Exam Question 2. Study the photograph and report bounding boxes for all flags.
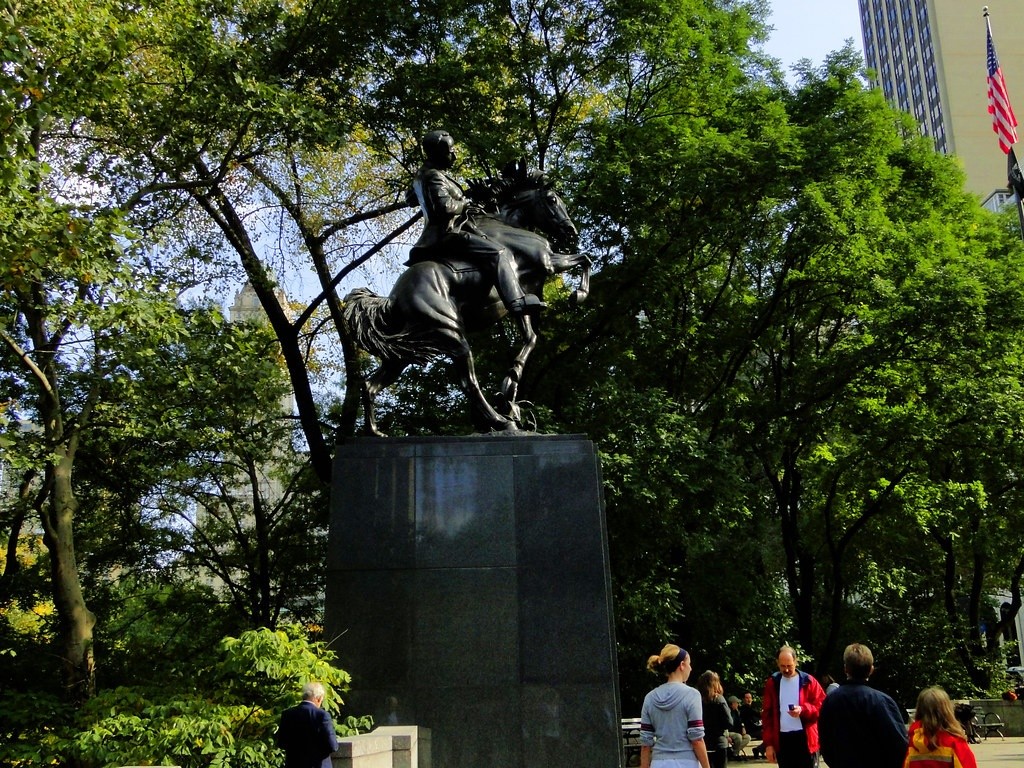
[987,26,1018,155]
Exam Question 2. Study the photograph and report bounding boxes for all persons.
[638,644,710,768]
[760,647,826,768]
[273,683,339,768]
[409,130,600,318]
[817,644,909,768]
[742,694,766,758]
[999,686,1024,701]
[697,670,733,768]
[954,700,981,744]
[903,688,977,768]
[822,674,839,695]
[728,696,751,756]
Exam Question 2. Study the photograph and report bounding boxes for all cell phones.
[789,705,794,710]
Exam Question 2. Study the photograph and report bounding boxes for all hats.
[728,695,742,703]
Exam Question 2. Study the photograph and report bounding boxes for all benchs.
[972,706,1005,744]
[621,717,641,768]
[906,708,916,722]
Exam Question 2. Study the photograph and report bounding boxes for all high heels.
[967,736,976,745]
[973,736,980,744]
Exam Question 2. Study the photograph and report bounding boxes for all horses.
[338,171,594,442]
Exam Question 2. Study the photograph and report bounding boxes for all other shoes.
[731,755,743,762]
[752,747,760,760]
[760,751,768,760]
